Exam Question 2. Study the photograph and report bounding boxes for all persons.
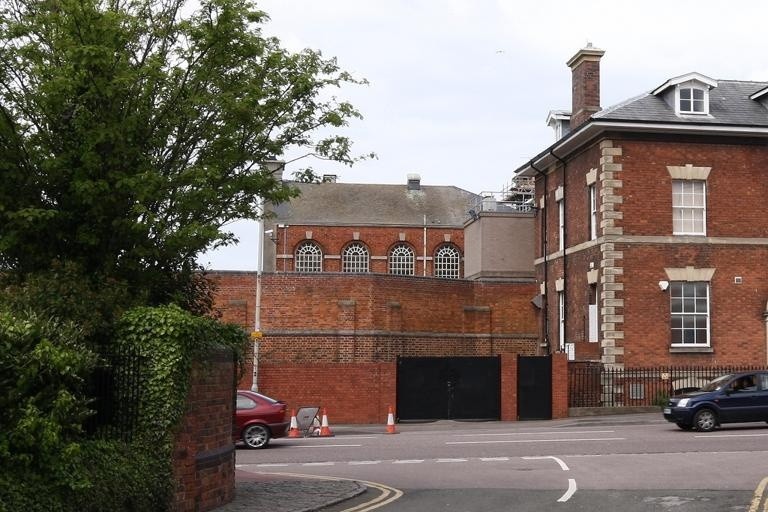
[740,376,752,388]
[731,380,739,389]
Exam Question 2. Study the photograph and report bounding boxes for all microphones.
[265,230,273,234]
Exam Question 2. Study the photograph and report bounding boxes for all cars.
[235,389,292,449]
[663,370,768,432]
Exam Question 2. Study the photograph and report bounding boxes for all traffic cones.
[313,411,320,432]
[385,406,400,434]
[288,408,300,437]
[318,407,334,437]
